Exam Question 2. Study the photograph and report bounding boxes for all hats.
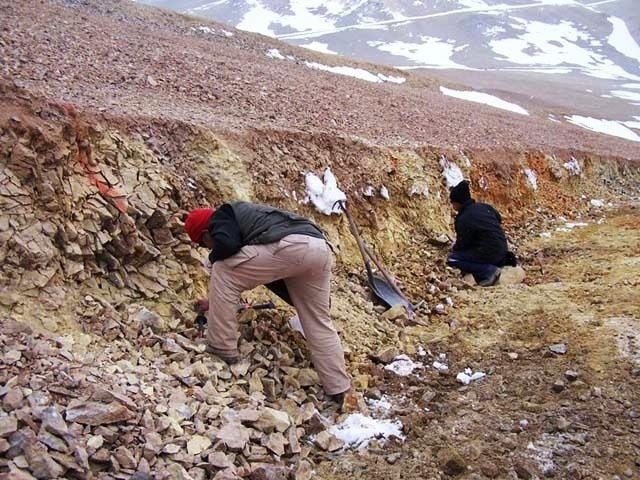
[450,181,473,204]
[185,208,214,243]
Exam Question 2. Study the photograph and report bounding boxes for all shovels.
[342,213,410,310]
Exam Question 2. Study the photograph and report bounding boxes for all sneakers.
[336,389,351,403]
[207,348,239,363]
[480,268,501,286]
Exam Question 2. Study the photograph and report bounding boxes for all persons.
[183,201,351,402]
[447,180,516,286]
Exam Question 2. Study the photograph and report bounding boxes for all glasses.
[198,235,208,248]
